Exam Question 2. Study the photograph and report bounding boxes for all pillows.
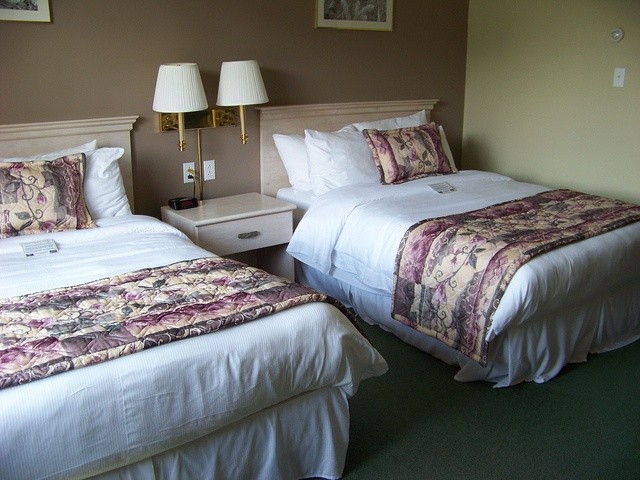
[270,125,370,189]
[3,138,100,162]
[82,146,134,227]
[0,153,97,239]
[437,126,458,174]
[361,123,463,182]
[353,108,427,134]
[303,128,385,191]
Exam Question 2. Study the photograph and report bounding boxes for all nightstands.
[160,191,296,286]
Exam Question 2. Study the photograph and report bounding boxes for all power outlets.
[182,162,196,185]
[202,159,216,181]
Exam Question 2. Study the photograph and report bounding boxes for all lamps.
[214,60,271,144]
[151,60,209,152]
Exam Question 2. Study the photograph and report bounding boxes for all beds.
[254,97,639,389]
[0,116,390,480]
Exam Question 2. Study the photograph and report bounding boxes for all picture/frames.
[0,0,52,25]
[311,0,395,32]
